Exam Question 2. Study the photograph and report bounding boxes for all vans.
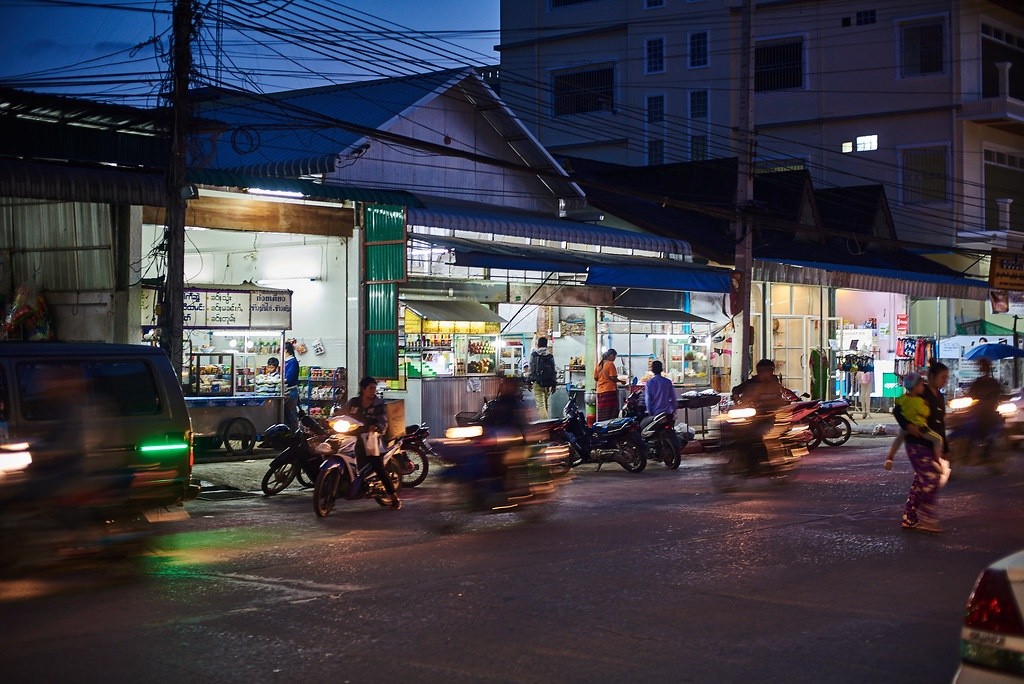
[0,338,193,520]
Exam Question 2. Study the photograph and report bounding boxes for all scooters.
[941,380,1009,460]
[725,398,827,470]
[454,393,581,478]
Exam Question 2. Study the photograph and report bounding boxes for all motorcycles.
[306,409,412,519]
[776,373,858,446]
[618,389,682,470]
[256,401,438,495]
[536,383,649,475]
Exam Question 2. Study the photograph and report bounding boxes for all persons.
[855,343,872,419]
[334,378,402,510]
[731,358,781,474]
[522,364,533,382]
[595,349,626,423]
[285,341,300,433]
[645,360,677,417]
[527,337,557,420]
[513,370,519,377]
[884,372,944,475]
[979,338,987,344]
[892,357,952,528]
[966,361,1012,415]
[258,356,280,375]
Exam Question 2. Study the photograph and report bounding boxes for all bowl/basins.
[200,348,214,353]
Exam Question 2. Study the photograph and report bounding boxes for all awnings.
[577,260,733,305]
[405,231,590,333]
[604,306,717,385]
[398,299,508,378]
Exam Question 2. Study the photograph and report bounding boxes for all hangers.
[898,335,932,341]
[836,353,873,360]
[895,356,912,361]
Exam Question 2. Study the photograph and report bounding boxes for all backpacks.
[532,350,554,387]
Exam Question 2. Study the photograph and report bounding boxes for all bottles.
[236,338,281,355]
[298,365,312,377]
[671,346,681,360]
[961,346,965,357]
[406,334,496,377]
[991,291,1008,312]
[940,388,947,404]
[958,377,975,393]
[1002,260,1024,270]
[569,357,584,370]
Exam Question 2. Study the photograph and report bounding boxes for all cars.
[995,385,1024,433]
[951,548,1024,683]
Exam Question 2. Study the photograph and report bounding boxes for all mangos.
[470,342,495,354]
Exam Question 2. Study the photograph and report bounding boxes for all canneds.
[407,342,416,351]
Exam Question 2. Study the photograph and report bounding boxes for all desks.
[677,396,722,438]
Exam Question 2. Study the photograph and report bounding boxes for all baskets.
[455,411,480,426]
[552,421,569,439]
[308,435,343,455]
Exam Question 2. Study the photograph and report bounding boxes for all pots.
[584,389,596,403]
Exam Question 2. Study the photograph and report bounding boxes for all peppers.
[966,589,1023,640]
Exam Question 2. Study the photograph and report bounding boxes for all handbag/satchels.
[360,431,381,457]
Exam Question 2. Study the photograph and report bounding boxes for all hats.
[903,373,921,392]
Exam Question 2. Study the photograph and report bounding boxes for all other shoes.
[390,500,402,510]
[884,460,893,472]
[902,520,943,533]
[932,460,944,474]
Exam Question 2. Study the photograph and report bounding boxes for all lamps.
[255,279,315,283]
[208,331,283,337]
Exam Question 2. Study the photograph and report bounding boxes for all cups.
[192,344,199,352]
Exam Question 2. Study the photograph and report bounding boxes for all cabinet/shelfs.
[666,343,706,380]
[262,366,346,417]
[399,332,524,374]
[187,352,258,397]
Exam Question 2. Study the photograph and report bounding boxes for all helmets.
[756,358,776,374]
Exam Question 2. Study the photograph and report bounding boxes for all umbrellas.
[962,344,1024,378]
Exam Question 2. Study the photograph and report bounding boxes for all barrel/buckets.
[707,419,721,436]
